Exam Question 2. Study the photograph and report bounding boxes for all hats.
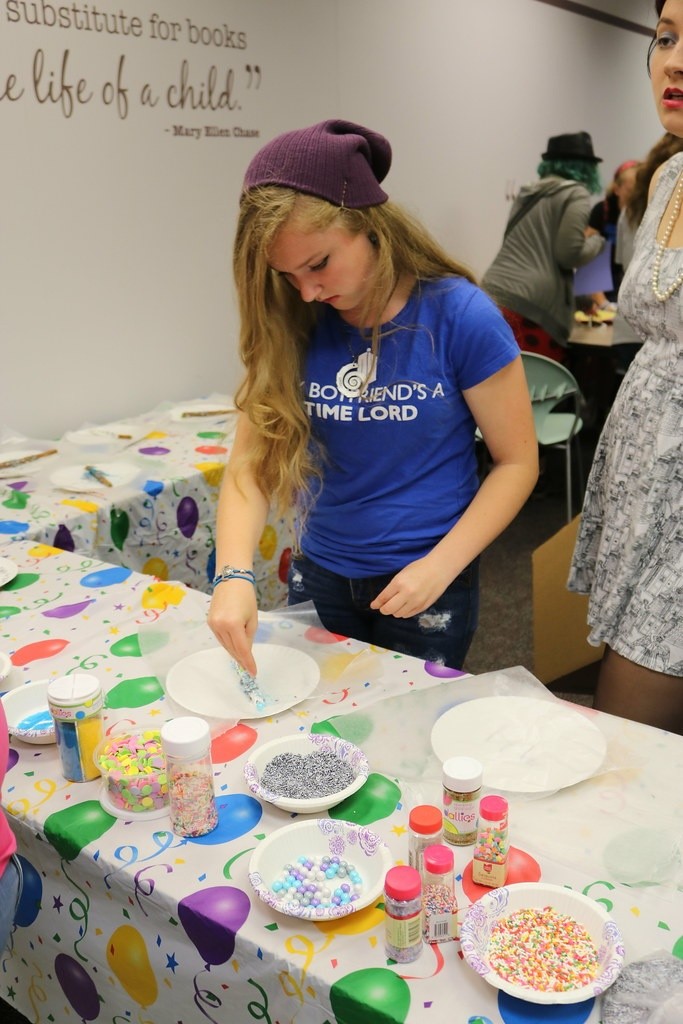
[243,117,392,207]
[543,131,602,163]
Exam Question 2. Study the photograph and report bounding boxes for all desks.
[568,302,643,384]
[0,397,683,1024]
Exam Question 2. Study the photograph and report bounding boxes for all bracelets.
[212,564,255,587]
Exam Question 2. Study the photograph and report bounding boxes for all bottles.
[162,716,219,836]
[47,674,103,782]
[442,756,484,847]
[473,796,510,889]
[408,805,443,869]
[382,865,422,963]
[423,845,458,944]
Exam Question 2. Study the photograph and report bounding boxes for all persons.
[206,119,540,677]
[482,130,682,495]
[564,4,683,739]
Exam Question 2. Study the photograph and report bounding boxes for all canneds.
[383,796,510,962]
[441,757,483,846]
[160,715,218,837]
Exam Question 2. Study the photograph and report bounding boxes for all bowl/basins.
[248,818,394,922]
[458,881,629,1004]
[0,652,12,682]
[244,733,370,814]
[2,679,59,745]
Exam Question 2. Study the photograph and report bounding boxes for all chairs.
[475,352,586,522]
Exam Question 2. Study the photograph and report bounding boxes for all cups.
[93,726,170,813]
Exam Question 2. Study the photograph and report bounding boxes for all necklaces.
[651,173,683,301]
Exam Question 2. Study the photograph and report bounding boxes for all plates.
[1,556,19,587]
[169,403,235,424]
[51,464,140,492]
[166,643,321,719]
[0,450,53,479]
[68,423,142,445]
[431,694,606,794]
